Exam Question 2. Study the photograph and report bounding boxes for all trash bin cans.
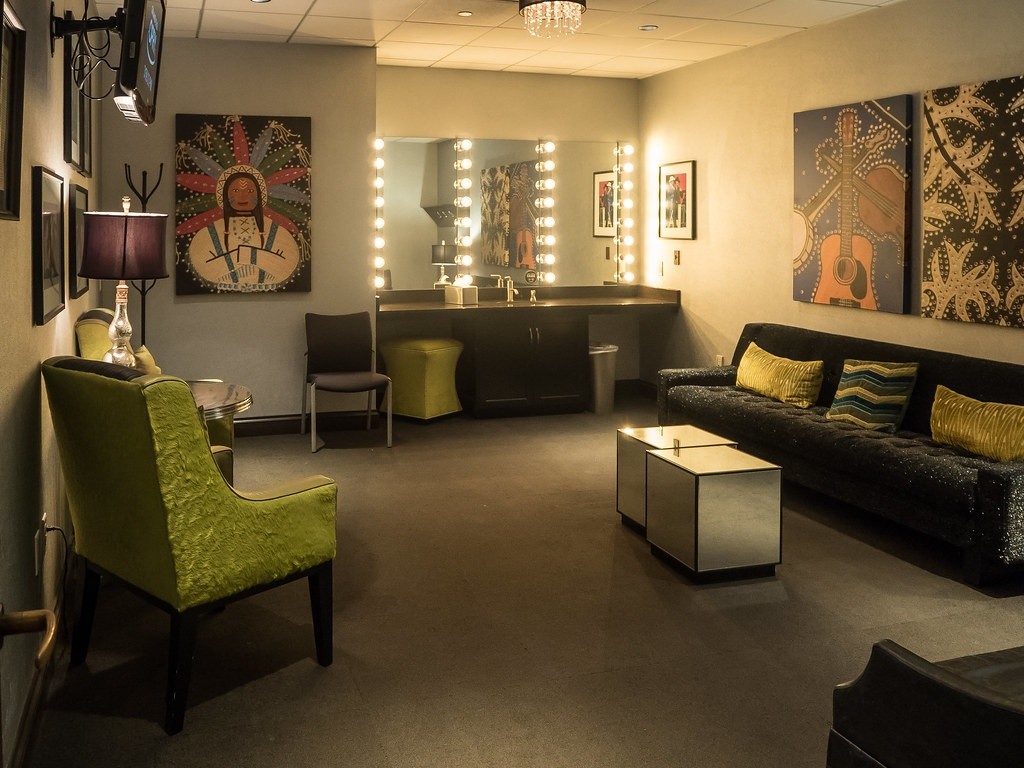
[589,339,619,415]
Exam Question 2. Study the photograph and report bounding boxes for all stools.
[378,337,467,420]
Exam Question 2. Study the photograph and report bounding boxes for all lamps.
[79,196,169,366]
[431,239,457,290]
[518,0,588,38]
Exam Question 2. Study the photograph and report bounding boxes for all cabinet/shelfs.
[452,311,591,410]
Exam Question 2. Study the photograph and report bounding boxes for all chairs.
[301,312,397,453]
[75,308,160,373]
[42,356,338,738]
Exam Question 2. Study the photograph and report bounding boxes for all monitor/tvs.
[113,0,166,127]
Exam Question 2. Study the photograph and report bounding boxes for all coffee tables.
[617,423,739,530]
[645,446,782,582]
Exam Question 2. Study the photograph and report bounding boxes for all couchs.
[825,640,1024,768]
[654,322,1024,596]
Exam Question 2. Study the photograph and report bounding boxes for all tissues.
[434,275,452,289]
[444,274,479,304]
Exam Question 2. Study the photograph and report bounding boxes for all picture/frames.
[656,161,697,239]
[592,172,619,236]
[0,1,103,326]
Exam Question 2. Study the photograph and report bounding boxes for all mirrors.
[381,136,619,290]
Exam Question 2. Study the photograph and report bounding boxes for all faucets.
[504,275,519,302]
[491,274,504,288]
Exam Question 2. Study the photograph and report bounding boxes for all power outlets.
[716,354,723,368]
[656,260,664,276]
[673,249,680,265]
[35,513,48,576]
[606,247,610,260]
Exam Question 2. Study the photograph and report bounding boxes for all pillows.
[825,358,918,434]
[737,341,825,409]
[932,385,1024,463]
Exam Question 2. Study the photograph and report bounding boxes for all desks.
[189,381,252,488]
[373,284,681,317]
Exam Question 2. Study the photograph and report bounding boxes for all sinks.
[496,301,545,305]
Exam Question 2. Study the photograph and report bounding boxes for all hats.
[604,184,608,189]
[675,177,680,184]
[607,182,611,187]
[669,176,675,182]
